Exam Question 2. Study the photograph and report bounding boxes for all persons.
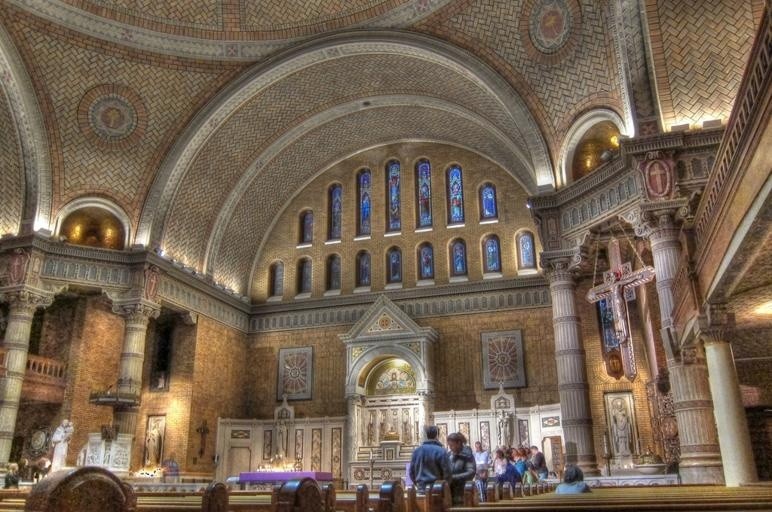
[51,418,74,472]
[614,401,631,454]
[590,271,644,338]
[273,410,289,460]
[555,466,591,494]
[498,399,511,446]
[408,425,549,507]
[145,422,161,465]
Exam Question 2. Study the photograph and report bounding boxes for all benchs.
[1,480,772,509]
